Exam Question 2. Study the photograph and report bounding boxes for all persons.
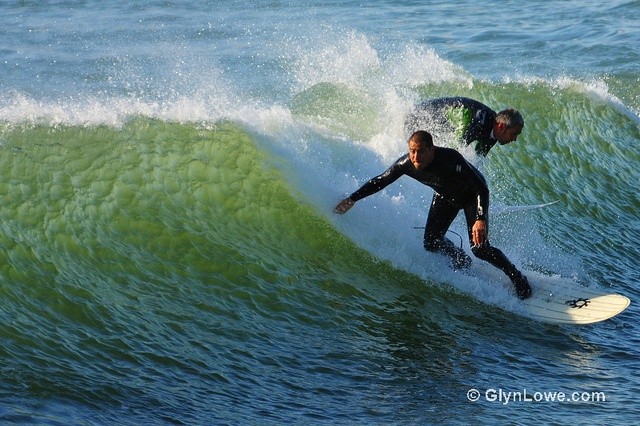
[332,131,532,301]
[404,97,523,169]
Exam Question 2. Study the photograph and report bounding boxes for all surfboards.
[407,245,631,325]
[490,199,560,213]
